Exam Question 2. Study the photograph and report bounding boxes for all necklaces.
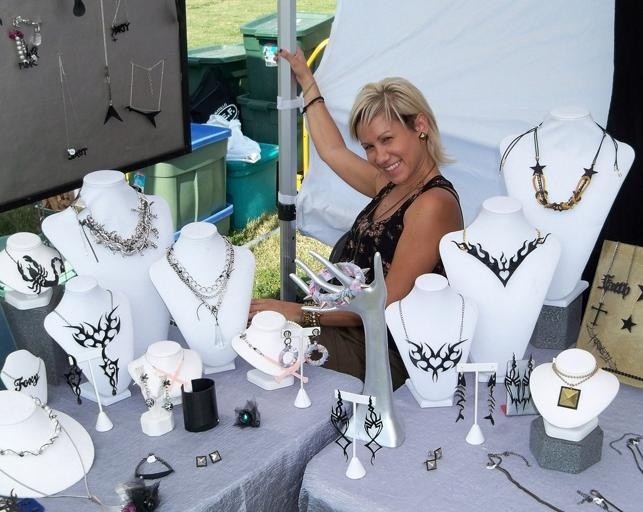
[551,361,601,411]
[450,223,551,288]
[399,293,464,384]
[501,118,624,212]
[348,159,437,267]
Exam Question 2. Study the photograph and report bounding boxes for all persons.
[246,41,462,391]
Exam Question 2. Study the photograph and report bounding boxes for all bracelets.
[301,95,325,115]
[299,298,321,327]
[303,80,316,97]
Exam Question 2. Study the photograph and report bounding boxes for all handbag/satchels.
[207,103,262,164]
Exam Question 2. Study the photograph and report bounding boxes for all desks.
[297,344,642,512]
[0,324,366,512]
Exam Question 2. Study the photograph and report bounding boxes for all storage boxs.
[125,8,336,242]
